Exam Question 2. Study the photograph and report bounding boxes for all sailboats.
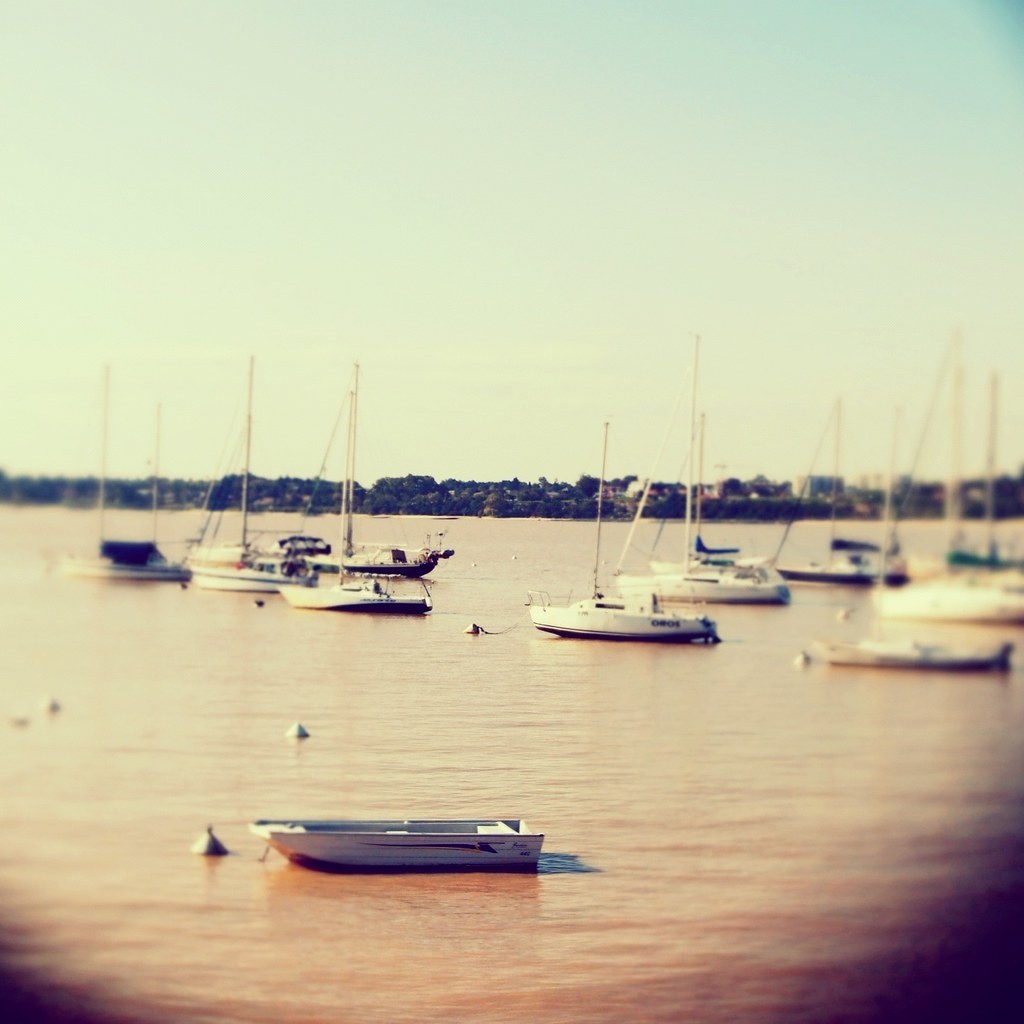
[50,350,454,611]
[531,335,1023,640]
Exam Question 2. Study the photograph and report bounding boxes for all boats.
[813,636,1014,673]
[247,815,545,871]
